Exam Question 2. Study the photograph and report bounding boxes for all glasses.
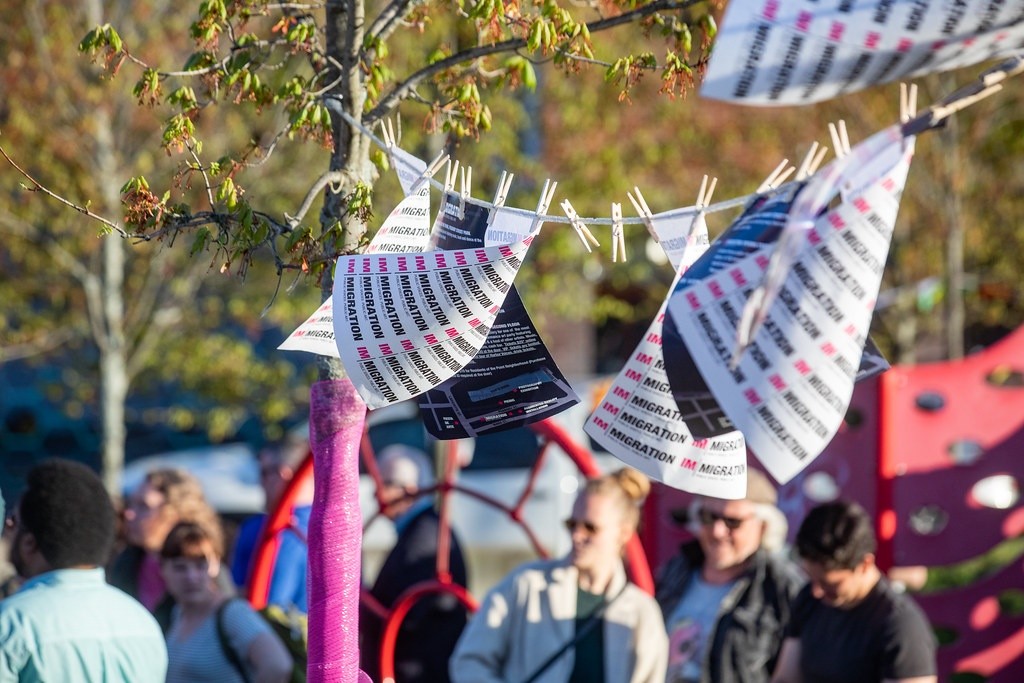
[698,508,755,528]
[565,518,599,533]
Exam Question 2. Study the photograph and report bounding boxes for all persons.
[963,281,1016,358]
[449,467,670,683]
[651,463,814,683]
[0,412,315,683]
[769,499,939,683]
[358,443,469,683]
